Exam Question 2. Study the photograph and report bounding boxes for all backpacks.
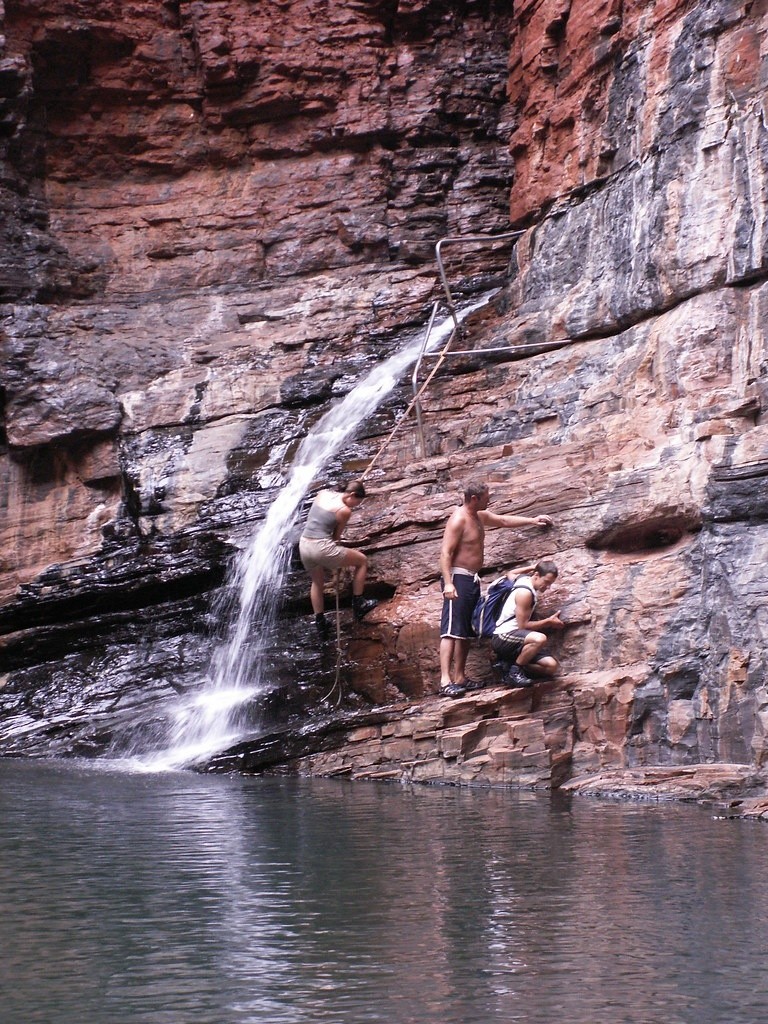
[471,574,535,638]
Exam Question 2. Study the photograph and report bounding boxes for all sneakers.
[315,617,333,634]
[504,665,533,687]
[352,597,378,620]
[493,662,509,683]
[438,685,464,697]
[453,679,485,690]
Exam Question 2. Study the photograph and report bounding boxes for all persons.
[491,561,564,686]
[299,481,378,628]
[439,481,552,697]
